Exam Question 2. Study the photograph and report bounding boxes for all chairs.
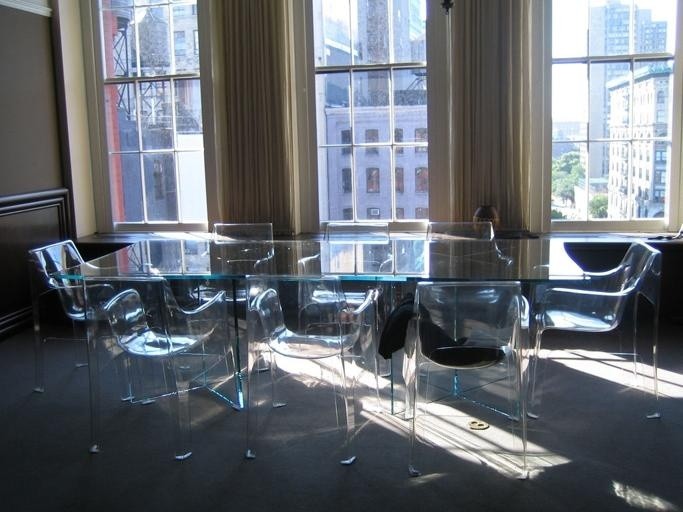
[524,240,665,426]
[27,239,157,414]
[242,273,388,468]
[293,221,396,381]
[398,279,534,476]
[78,275,247,465]
[414,221,518,393]
[181,220,281,377]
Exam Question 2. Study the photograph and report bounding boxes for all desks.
[46,235,534,429]
